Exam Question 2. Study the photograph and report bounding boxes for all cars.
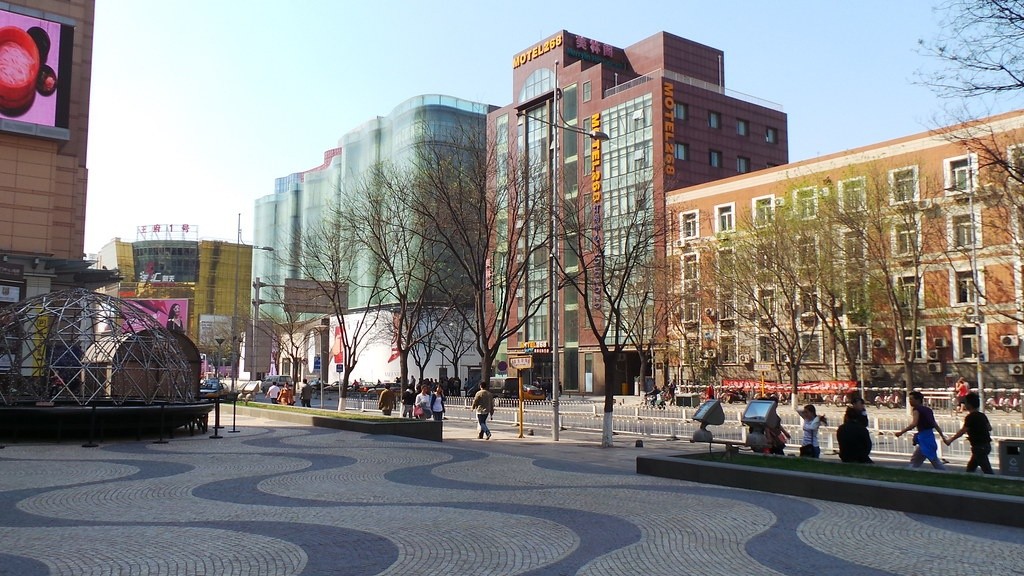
[516,59,611,441]
[200,378,227,390]
[308,380,401,400]
[523,385,545,399]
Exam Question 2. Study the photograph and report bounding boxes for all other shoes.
[486,433,491,440]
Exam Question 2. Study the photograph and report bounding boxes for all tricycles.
[640,394,666,410]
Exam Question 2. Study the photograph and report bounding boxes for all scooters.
[725,387,747,404]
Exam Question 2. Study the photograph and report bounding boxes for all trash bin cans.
[676,393,700,408]
[998,439,1024,477]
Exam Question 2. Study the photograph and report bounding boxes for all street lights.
[439,347,446,368]
[944,148,984,413]
[216,338,226,392]
[220,214,274,394]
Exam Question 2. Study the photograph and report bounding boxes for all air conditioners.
[963,303,978,317]
[705,332,713,340]
[676,239,686,248]
[785,301,795,310]
[1008,363,1024,375]
[739,354,751,364]
[707,350,716,358]
[871,338,887,349]
[1000,335,1019,347]
[870,368,884,378]
[698,360,708,369]
[933,337,947,348]
[927,363,941,373]
[926,349,938,361]
[704,307,715,316]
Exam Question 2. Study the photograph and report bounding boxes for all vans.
[467,377,518,398]
[261,375,293,393]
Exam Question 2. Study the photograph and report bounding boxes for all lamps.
[35,258,39,263]
[244,381,259,401]
[742,399,782,448]
[691,400,725,443]
[3,256,8,261]
[238,382,248,401]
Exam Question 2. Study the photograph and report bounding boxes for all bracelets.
[941,436,946,438]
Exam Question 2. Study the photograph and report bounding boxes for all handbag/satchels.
[800,444,814,457]
[414,405,423,415]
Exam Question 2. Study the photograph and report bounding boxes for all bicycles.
[690,391,731,403]
[986,393,1023,412]
[874,390,903,409]
[753,392,791,404]
[822,389,849,407]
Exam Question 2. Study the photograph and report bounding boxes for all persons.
[754,389,787,403]
[378,383,398,417]
[532,375,562,402]
[746,396,790,455]
[265,380,295,406]
[48,369,63,397]
[377,375,470,397]
[836,390,875,464]
[987,389,1020,413]
[430,383,447,421]
[895,392,948,471]
[470,382,494,439]
[955,376,969,413]
[821,391,848,406]
[316,377,322,396]
[795,402,827,458]
[165,303,184,331]
[873,391,903,408]
[401,385,416,419]
[946,392,996,475]
[681,382,746,403]
[299,378,313,408]
[645,376,676,409]
[352,378,360,391]
[414,383,432,419]
